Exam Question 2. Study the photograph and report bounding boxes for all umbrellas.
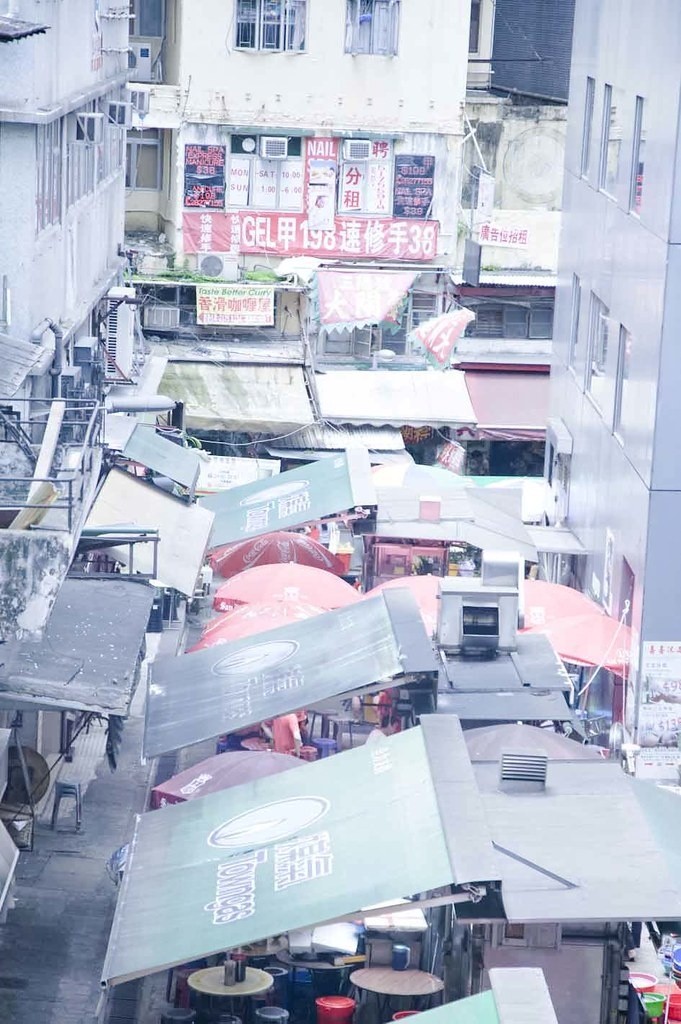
[518,580,632,676]
[463,722,604,762]
[184,530,440,654]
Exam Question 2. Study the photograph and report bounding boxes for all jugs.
[391,945,411,971]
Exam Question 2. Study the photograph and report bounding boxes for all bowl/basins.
[663,993,681,1021]
[641,992,667,1017]
[669,947,681,990]
[628,972,658,993]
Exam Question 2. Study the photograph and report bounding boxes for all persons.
[366,715,399,743]
[262,714,302,757]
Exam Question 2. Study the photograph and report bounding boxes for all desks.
[187,966,275,1024]
[275,946,356,1024]
[224,937,289,968]
[349,966,444,1024]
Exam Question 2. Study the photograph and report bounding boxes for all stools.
[160,1008,197,1024]
[315,996,356,1024]
[254,1005,289,1024]
[208,1014,242,1024]
[311,737,338,759]
[310,709,338,740]
[262,965,289,1010]
[327,715,354,749]
[51,776,83,832]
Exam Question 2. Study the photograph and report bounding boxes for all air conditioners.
[73,336,98,367]
[198,251,239,282]
[58,364,82,398]
[128,43,151,82]
[343,139,373,162]
[259,136,288,160]
[106,101,134,128]
[131,90,150,114]
[105,287,134,380]
[75,112,105,145]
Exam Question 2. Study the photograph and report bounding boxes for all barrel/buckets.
[392,1010,421,1021]
[315,996,356,1024]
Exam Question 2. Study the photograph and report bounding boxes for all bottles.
[224,960,236,986]
[231,954,246,982]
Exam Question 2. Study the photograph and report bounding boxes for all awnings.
[1,572,156,720]
[196,445,377,550]
[383,966,561,1024]
[375,483,538,564]
[524,525,585,554]
[471,762,680,925]
[81,469,216,598]
[437,633,573,722]
[100,711,581,990]
[149,361,551,443]
[141,586,439,766]
